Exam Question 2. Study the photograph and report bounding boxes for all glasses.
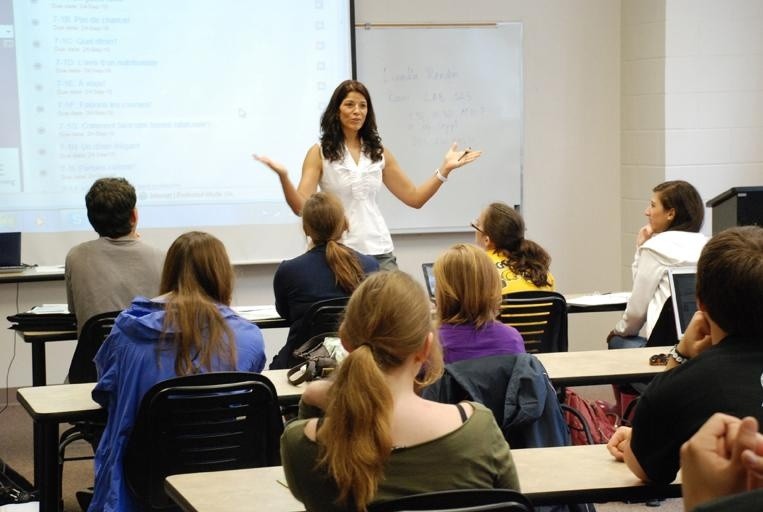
[469,219,483,233]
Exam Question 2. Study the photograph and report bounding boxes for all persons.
[606,180,713,415]
[253,80,482,271]
[268,192,379,369]
[280,270,521,512]
[607,223,763,486]
[84,230,267,512]
[678,411,763,512]
[471,203,556,354]
[424,244,526,364]
[63,179,164,454]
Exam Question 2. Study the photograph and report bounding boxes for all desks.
[164,443,682,512]
[13,288,633,490]
[0,265,68,282]
[16,344,675,511]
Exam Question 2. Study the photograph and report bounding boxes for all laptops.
[0,232,21,266]
[422,264,436,303]
[668,266,698,340]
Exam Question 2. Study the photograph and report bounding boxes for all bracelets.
[667,344,691,365]
[434,168,448,182]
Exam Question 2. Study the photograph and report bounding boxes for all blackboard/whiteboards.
[355,21,525,235]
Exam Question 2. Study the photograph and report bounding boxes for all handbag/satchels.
[288,331,351,386]
[556,386,617,444]
[0,458,39,507]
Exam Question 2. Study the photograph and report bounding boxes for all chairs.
[298,295,352,335]
[57,309,124,469]
[420,351,576,449]
[618,295,679,428]
[499,291,569,403]
[122,370,284,511]
[356,488,535,510]
